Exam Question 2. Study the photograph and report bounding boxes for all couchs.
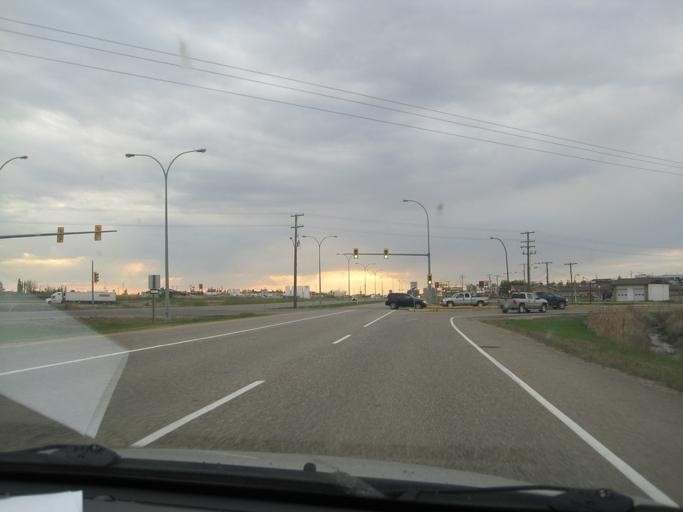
[428,274,432,285]
[353,249,359,258]
[94,272,98,282]
[384,249,388,259]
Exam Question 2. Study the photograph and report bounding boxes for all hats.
[535,291,568,309]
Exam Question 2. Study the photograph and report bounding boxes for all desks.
[491,237,509,297]
[0,157,27,172]
[403,199,432,306]
[290,237,295,247]
[124,149,206,319]
[302,235,338,298]
[574,274,579,303]
[337,253,383,299]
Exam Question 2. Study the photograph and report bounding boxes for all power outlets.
[496,292,548,313]
[441,291,489,307]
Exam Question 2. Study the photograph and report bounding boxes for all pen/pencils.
[384,293,427,310]
